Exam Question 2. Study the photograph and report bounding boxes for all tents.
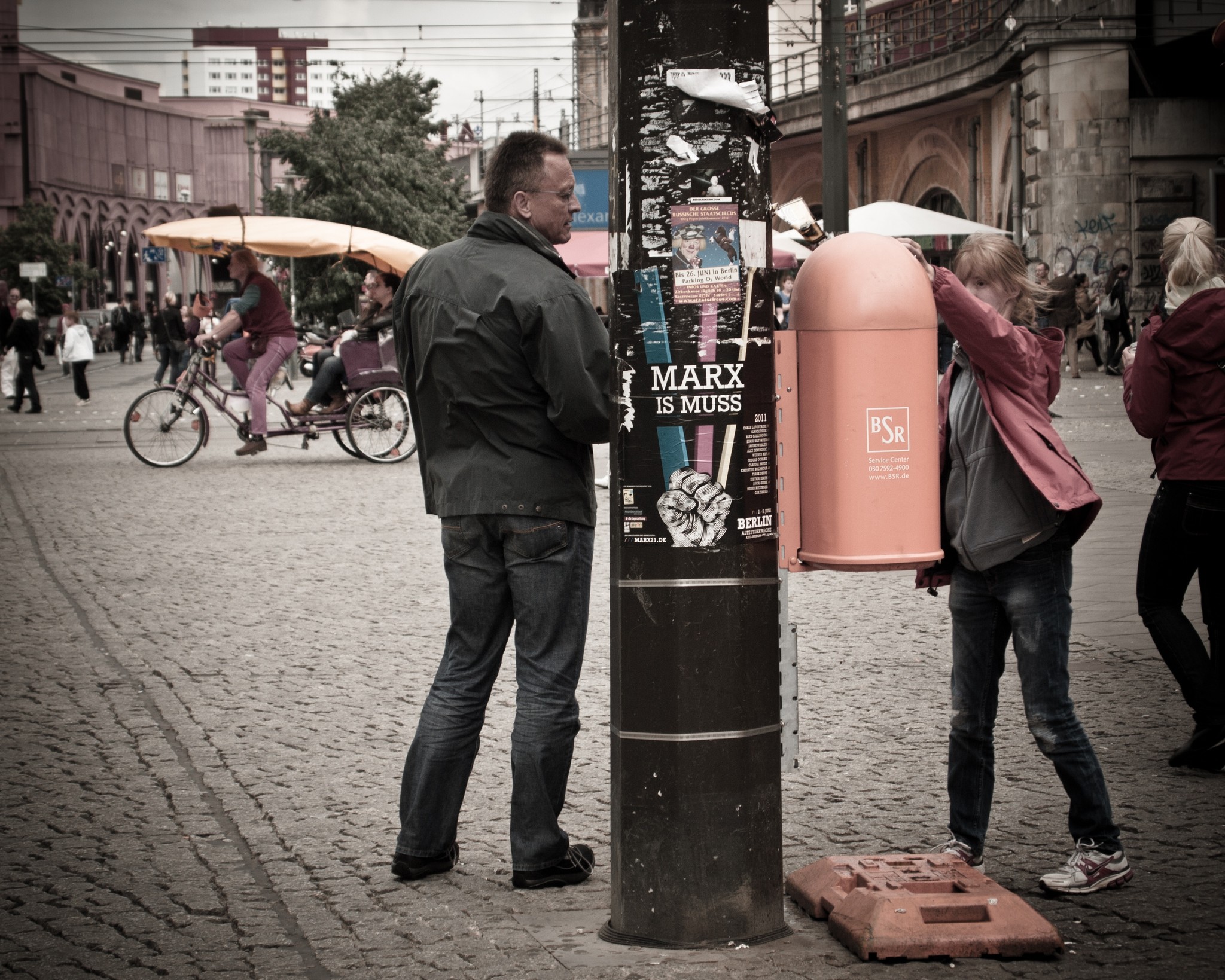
[557,198,1014,280]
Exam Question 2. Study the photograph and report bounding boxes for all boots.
[320,384,348,415]
[285,399,316,416]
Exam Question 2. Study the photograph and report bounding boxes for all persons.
[0,269,399,455]
[394,129,616,889]
[775,219,1225,894]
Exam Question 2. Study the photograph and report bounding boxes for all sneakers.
[1040,836,1133,894]
[512,844,596,888]
[392,840,460,880]
[929,827,986,875]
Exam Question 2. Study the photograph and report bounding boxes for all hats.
[192,294,213,319]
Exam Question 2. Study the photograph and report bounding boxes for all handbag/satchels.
[1100,278,1121,320]
[169,339,187,355]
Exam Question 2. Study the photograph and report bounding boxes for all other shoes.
[25,409,39,413]
[77,398,90,405]
[236,440,267,456]
[1066,365,1082,372]
[1098,364,1106,372]
[1108,364,1122,375]
[154,381,159,387]
[1169,717,1225,773]
[7,406,19,413]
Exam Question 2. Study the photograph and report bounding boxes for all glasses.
[372,282,387,289]
[524,188,573,200]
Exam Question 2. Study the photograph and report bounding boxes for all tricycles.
[123,216,429,469]
